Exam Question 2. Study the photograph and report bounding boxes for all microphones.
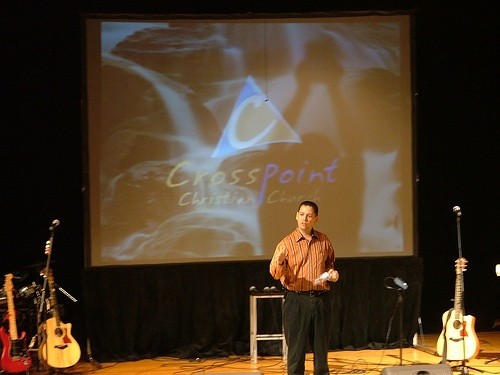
[453,206,461,216]
[49,219,60,230]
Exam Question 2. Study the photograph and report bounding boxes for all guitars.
[435,257,481,361]
[0,272,33,374]
[36,268,82,369]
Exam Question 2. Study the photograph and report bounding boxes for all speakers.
[379,364,453,375]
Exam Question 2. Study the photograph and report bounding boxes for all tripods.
[451,215,485,375]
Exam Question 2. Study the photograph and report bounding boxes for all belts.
[291,290,328,297]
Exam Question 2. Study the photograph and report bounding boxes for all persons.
[269,201,339,375]
